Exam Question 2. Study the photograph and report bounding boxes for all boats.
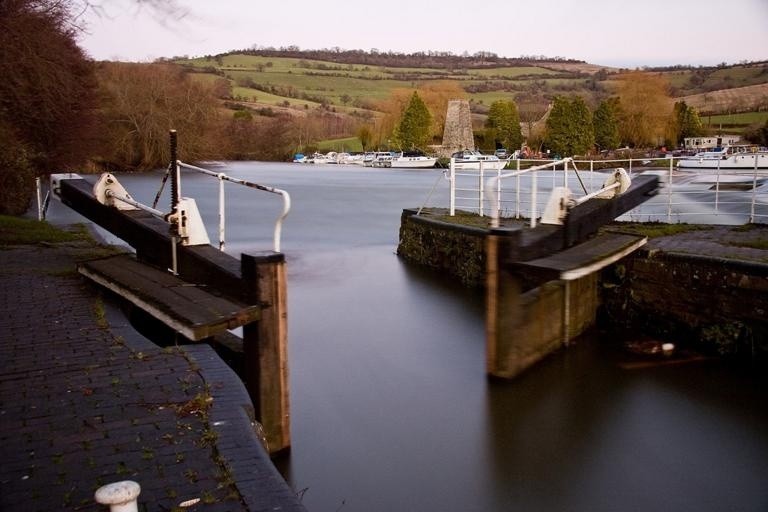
[448,150,511,170]
[291,136,440,170]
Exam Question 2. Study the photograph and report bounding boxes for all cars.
[495,150,508,159]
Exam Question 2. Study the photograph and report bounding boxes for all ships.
[675,144,767,168]
